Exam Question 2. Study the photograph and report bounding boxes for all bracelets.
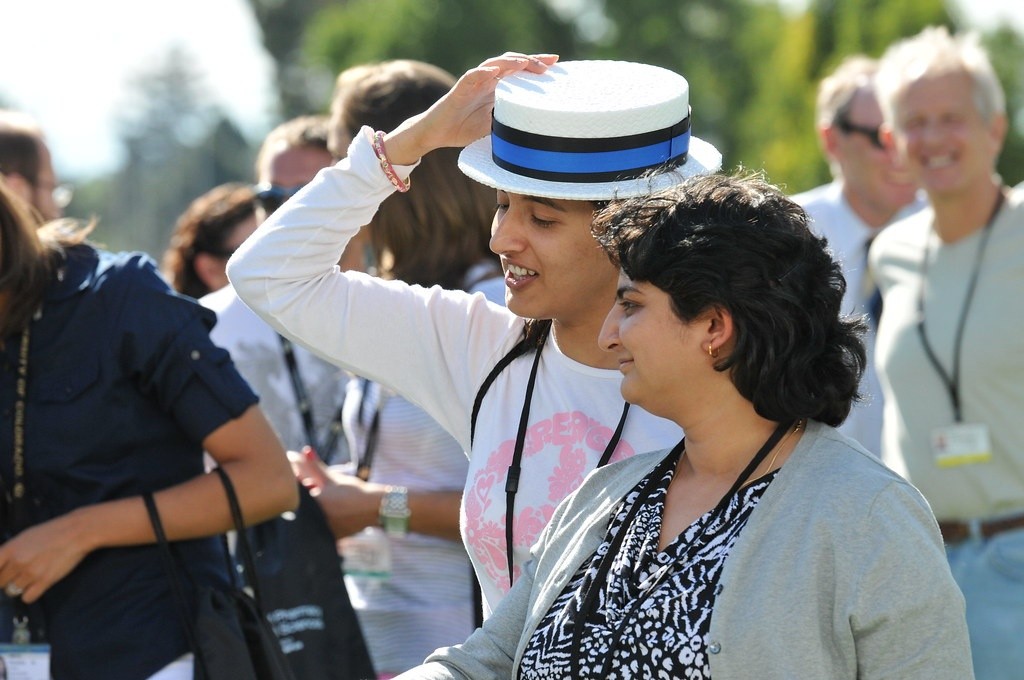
[374,129,412,194]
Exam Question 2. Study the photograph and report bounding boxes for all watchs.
[380,483,412,541]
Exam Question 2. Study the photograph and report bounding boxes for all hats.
[456,59,724,201]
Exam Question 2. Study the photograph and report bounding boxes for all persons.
[0,118,64,236]
[281,55,538,680]
[158,178,261,300]
[861,20,1024,679]
[221,44,726,617]
[0,173,303,679]
[185,108,382,599]
[385,171,979,679]
[778,50,937,466]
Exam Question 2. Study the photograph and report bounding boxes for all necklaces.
[664,418,804,497]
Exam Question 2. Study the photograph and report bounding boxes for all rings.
[4,579,23,597]
[493,76,501,81]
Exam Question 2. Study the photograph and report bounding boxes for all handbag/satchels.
[142,465,296,680]
[234,478,376,680]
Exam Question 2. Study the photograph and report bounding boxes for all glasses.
[252,183,307,218]
[38,179,75,209]
[834,114,883,151]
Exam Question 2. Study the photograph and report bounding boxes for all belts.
[937,515,1023,544]
